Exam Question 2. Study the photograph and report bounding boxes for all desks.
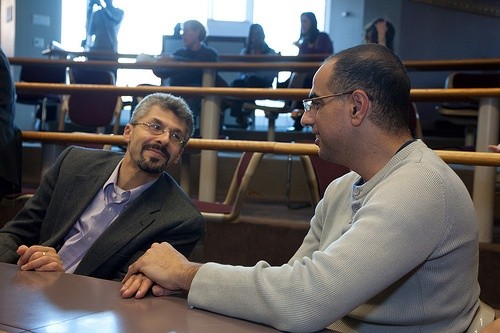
[0,259,346,333]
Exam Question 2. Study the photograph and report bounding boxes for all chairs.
[17,52,500,333]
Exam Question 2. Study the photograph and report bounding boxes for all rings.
[42,251,45,255]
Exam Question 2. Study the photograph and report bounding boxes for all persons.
[288,12,334,132]
[120,43,481,333]
[228,23,276,129]
[85,0,124,81]
[363,19,395,53]
[169,20,218,116]
[0,93,204,280]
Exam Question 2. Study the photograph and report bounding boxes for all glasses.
[303,90,374,115]
[130,122,185,144]
